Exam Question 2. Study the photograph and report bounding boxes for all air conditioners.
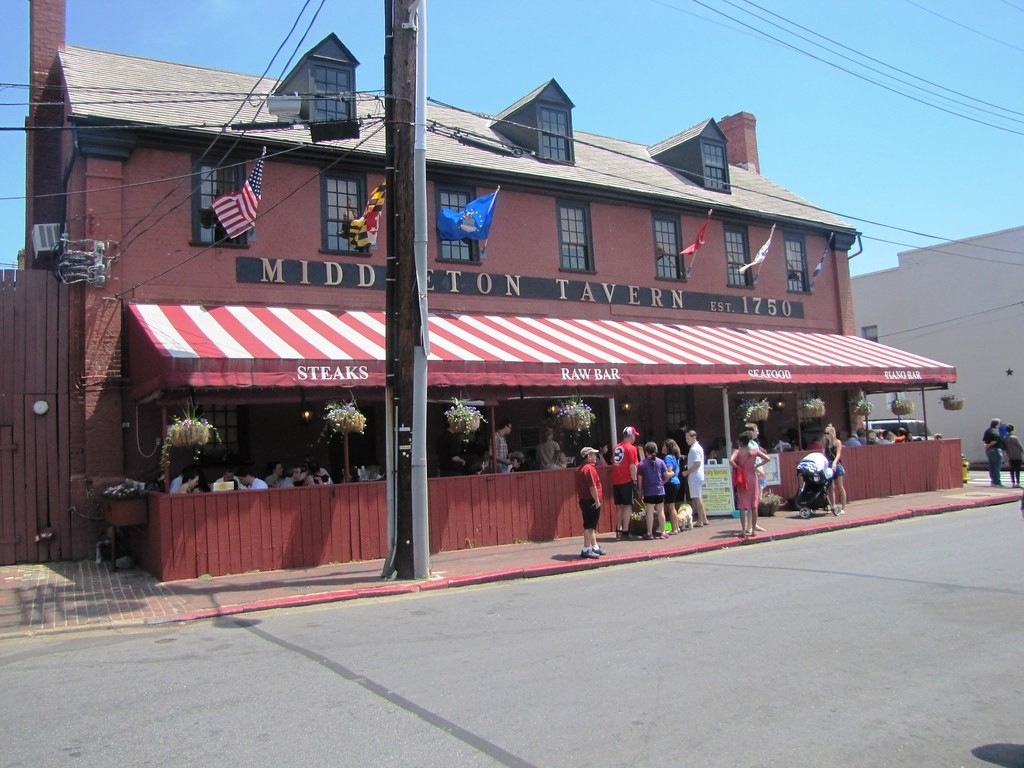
[32,223,61,259]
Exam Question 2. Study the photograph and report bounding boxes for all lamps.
[775,393,786,410]
[619,391,634,416]
[299,386,313,423]
[548,399,559,416]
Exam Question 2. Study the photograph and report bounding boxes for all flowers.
[936,393,963,404]
[444,396,489,452]
[758,489,783,506]
[103,483,146,498]
[888,399,914,406]
[160,397,222,470]
[732,397,773,423]
[304,398,367,449]
[550,399,596,447]
[799,391,827,417]
[629,488,658,520]
[850,400,875,415]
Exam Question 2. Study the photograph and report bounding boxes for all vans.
[858,417,934,441]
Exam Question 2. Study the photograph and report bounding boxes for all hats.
[623,427,639,436]
[580,447,599,457]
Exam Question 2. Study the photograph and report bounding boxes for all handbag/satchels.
[841,466,846,475]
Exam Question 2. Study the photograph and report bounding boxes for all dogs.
[677,503,694,531]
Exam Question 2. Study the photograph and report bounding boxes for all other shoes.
[755,526,767,532]
[678,528,680,533]
[619,533,639,540]
[991,483,1003,487]
[1012,483,1023,488]
[667,529,678,535]
[740,531,757,537]
[660,533,669,538]
[643,533,654,540]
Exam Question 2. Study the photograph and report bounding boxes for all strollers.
[791,453,843,518]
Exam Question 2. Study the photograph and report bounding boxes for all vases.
[944,399,963,410]
[758,504,778,516]
[800,404,825,417]
[560,414,591,431]
[449,415,480,433]
[100,494,147,526]
[740,408,768,422]
[622,513,659,536]
[891,405,915,414]
[851,404,871,415]
[329,414,364,432]
[170,426,208,447]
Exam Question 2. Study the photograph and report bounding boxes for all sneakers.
[592,546,606,555]
[581,549,599,558]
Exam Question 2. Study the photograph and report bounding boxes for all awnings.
[126,303,957,402]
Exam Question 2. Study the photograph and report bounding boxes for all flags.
[680,216,709,256]
[737,238,770,274]
[811,238,830,276]
[437,191,496,241]
[212,158,264,238]
[343,182,388,252]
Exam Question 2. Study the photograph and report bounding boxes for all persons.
[1003,425,1024,487]
[614,425,639,540]
[682,430,709,528]
[662,438,682,537]
[637,442,669,541]
[822,426,847,515]
[169,461,360,493]
[443,420,921,476]
[577,447,610,558]
[983,418,1004,488]
[729,423,772,535]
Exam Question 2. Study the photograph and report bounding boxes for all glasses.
[824,432,829,435]
